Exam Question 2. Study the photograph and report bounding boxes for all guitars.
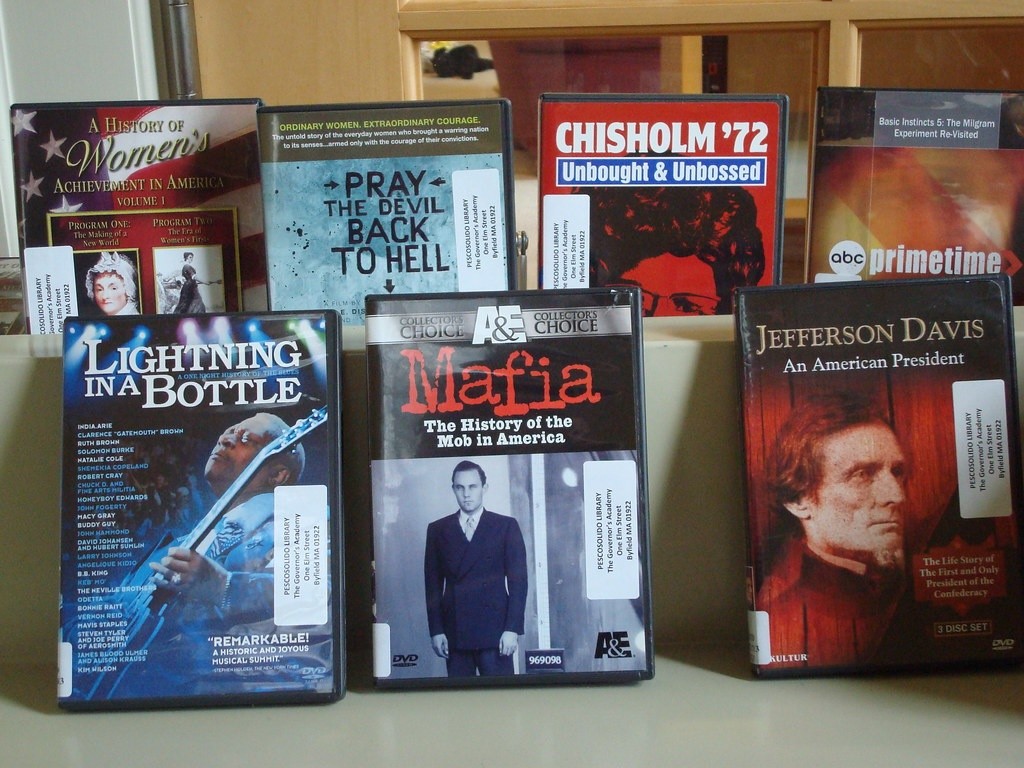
[74,403,329,701]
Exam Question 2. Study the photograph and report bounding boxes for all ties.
[464,517,475,542]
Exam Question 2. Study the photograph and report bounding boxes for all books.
[801,84,1022,304]
[253,96,520,323]
[8,98,272,340]
[733,272,1024,679]
[534,91,785,315]
[363,290,654,686]
[56,307,351,704]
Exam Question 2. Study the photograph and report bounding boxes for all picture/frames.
[361,285,658,687]
[802,85,1023,306]
[9,95,270,335]
[59,309,347,712]
[731,272,1023,681]
[254,95,517,350]
[537,92,791,316]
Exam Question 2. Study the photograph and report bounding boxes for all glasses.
[603,279,718,316]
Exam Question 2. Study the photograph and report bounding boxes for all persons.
[63,413,306,696]
[421,460,528,678]
[753,383,935,669]
